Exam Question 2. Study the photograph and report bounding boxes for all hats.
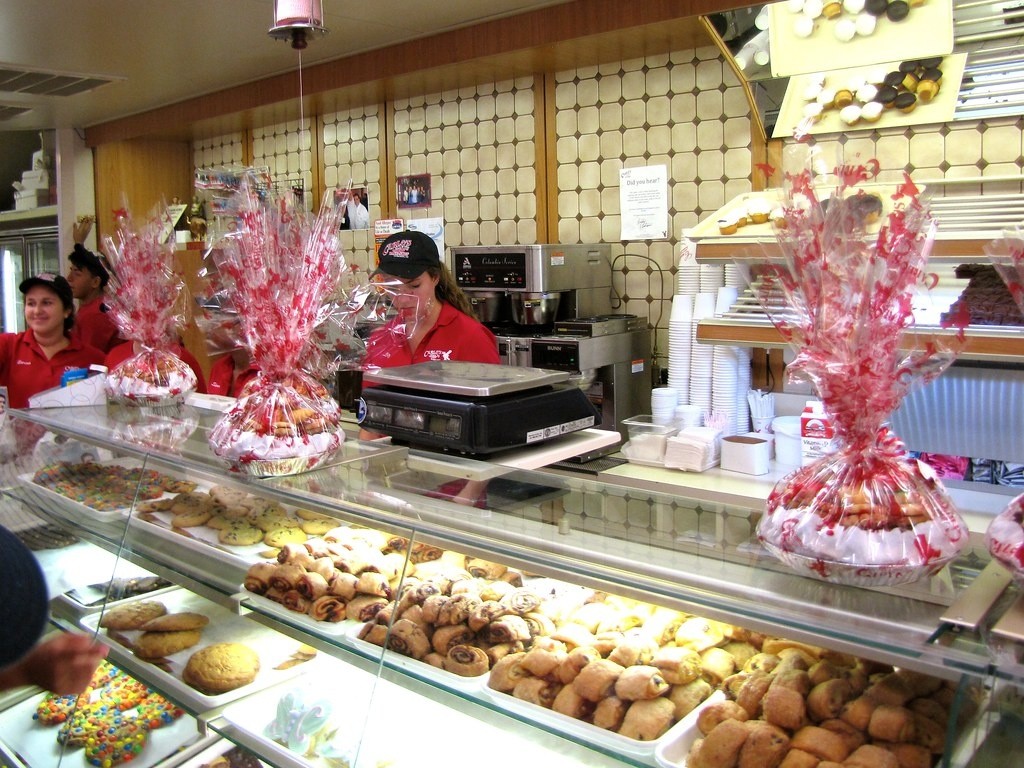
[195,289,240,315]
[75,243,111,286]
[19,272,73,307]
[368,230,440,280]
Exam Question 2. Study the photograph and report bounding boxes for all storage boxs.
[14,151,54,209]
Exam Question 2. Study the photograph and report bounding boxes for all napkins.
[667,427,726,470]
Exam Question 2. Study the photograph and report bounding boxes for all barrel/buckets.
[773,416,801,465]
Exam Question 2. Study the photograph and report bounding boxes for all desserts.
[717,196,791,234]
[788,0,946,127]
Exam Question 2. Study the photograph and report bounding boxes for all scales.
[358,361,604,454]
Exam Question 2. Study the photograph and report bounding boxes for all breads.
[241,534,983,768]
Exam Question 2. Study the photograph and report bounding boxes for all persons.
[0,216,270,457]
[340,186,428,230]
[0,633,109,697]
[912,453,1024,487]
[943,263,1024,327]
[363,231,504,507]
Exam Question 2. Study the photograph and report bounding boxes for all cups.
[668,229,751,433]
[752,415,776,460]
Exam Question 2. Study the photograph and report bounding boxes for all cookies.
[31,598,260,768]
[33,461,199,512]
[105,349,196,399]
[207,372,345,462]
[756,468,969,564]
[135,481,342,558]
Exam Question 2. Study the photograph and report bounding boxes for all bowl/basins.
[465,291,503,323]
[511,293,561,324]
